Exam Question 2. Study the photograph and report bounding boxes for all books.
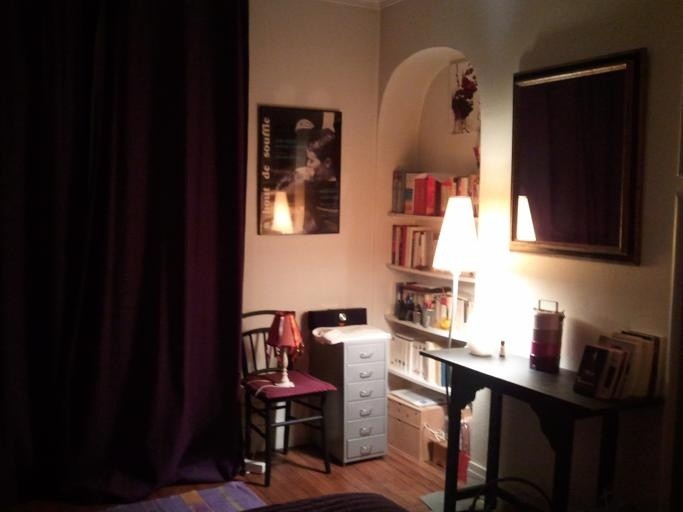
[389,169,480,409]
[572,328,665,401]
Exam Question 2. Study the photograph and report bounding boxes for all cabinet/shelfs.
[311,325,394,465]
[384,211,480,397]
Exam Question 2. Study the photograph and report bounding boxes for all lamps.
[264,310,307,388]
[431,195,485,347]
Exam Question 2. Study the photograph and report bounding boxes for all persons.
[262,147,338,234]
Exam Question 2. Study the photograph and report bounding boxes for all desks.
[419,345,656,511]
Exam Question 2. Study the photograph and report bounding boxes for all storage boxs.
[387,387,448,462]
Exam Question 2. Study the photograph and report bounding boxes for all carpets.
[111,477,268,511]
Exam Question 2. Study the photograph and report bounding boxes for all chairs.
[237,309,341,487]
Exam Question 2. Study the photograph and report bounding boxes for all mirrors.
[507,43,648,270]
